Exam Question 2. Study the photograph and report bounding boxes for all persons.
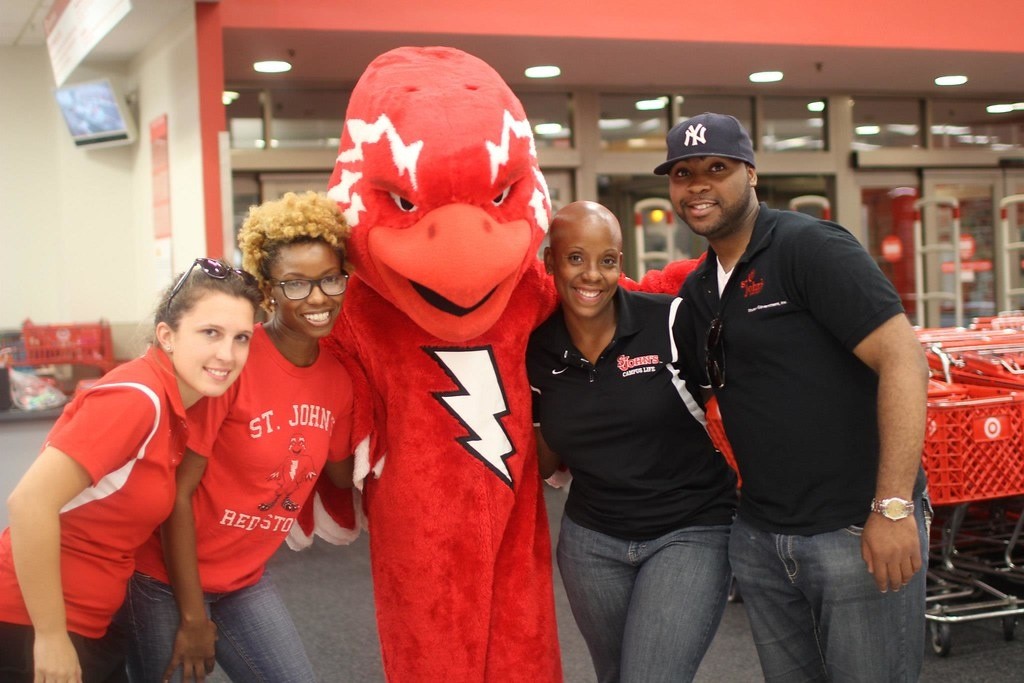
[115,192,357,682]
[524,200,734,683]
[0,256,262,683]
[653,112,937,683]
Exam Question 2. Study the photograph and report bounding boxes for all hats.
[652,111,756,175]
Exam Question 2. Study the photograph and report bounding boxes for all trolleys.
[909,308,1024,656]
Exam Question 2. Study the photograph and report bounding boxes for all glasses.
[704,313,726,388]
[166,257,258,311]
[270,269,348,300]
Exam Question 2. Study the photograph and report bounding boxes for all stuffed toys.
[280,46,715,683]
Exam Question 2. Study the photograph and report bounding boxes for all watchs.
[870,495,914,521]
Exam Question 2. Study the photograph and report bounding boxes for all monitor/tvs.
[54,76,137,150]
